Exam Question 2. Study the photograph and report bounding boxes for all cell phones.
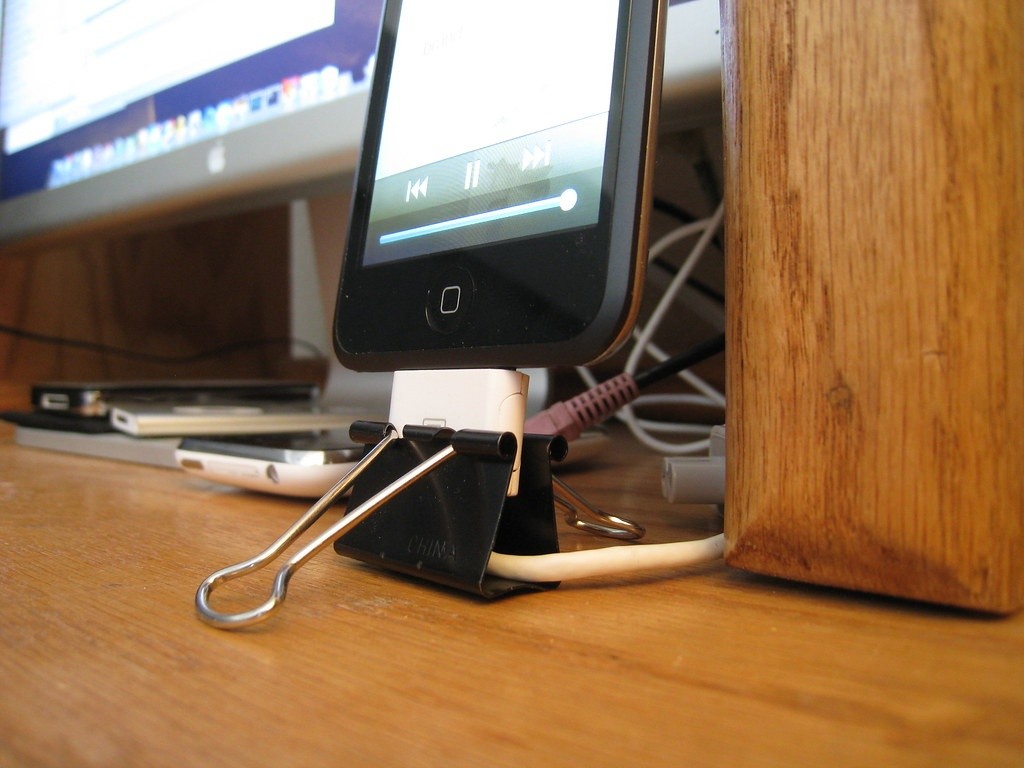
[175,427,364,499]
[331,0,670,372]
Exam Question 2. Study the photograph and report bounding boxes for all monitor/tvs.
[0,0,387,358]
